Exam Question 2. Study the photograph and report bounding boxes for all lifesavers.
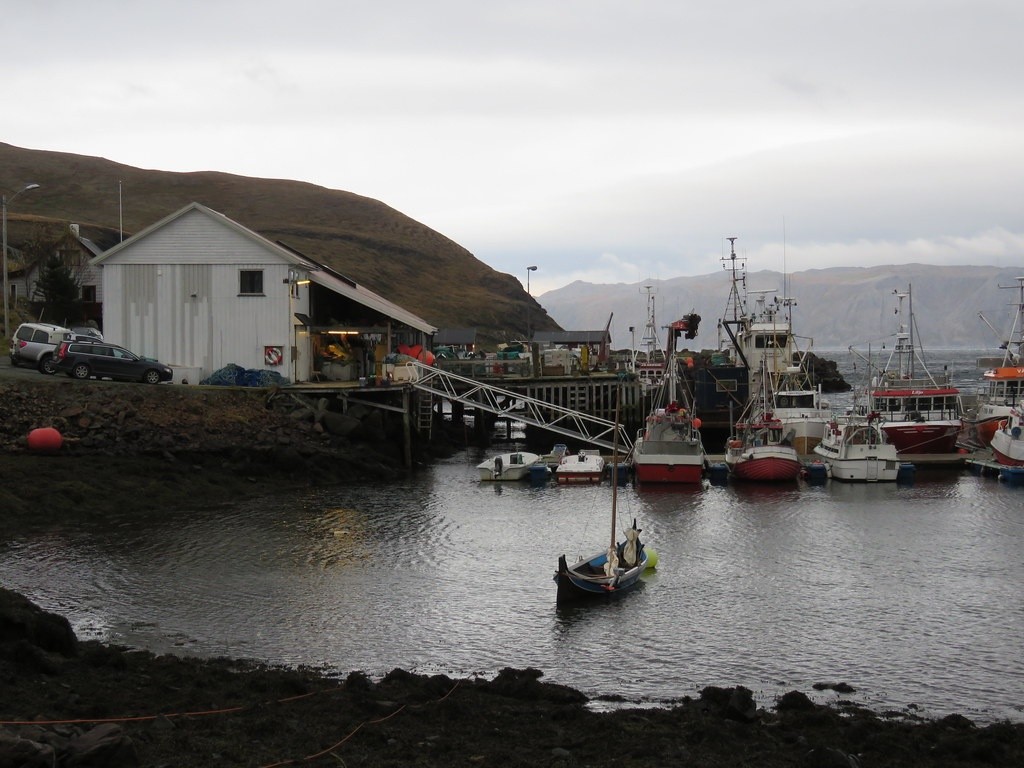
[265,347,282,365]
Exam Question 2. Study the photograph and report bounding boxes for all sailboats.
[552,387,646,603]
[725,330,803,484]
[633,325,704,484]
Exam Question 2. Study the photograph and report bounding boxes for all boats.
[555,451,604,484]
[745,278,1024,465]
[813,342,899,481]
[527,454,548,483]
[476,443,539,481]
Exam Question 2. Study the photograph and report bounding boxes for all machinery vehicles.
[595,312,613,358]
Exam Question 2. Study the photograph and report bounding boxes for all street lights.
[527,266,538,352]
[629,326,634,370]
[2,184,40,339]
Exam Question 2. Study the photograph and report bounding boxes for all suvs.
[8,322,173,384]
